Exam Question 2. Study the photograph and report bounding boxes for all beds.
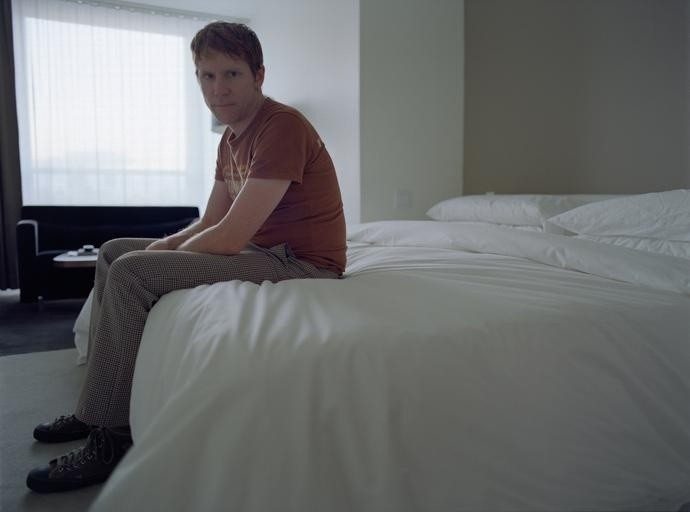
[61,191,690,512]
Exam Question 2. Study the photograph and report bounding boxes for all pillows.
[425,189,690,259]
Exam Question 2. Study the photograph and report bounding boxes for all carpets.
[0,289,88,356]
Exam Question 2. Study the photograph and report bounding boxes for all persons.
[26,23,346,493]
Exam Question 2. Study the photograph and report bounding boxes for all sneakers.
[26,429,126,494]
[33,414,98,444]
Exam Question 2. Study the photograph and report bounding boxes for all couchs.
[16,205,200,302]
